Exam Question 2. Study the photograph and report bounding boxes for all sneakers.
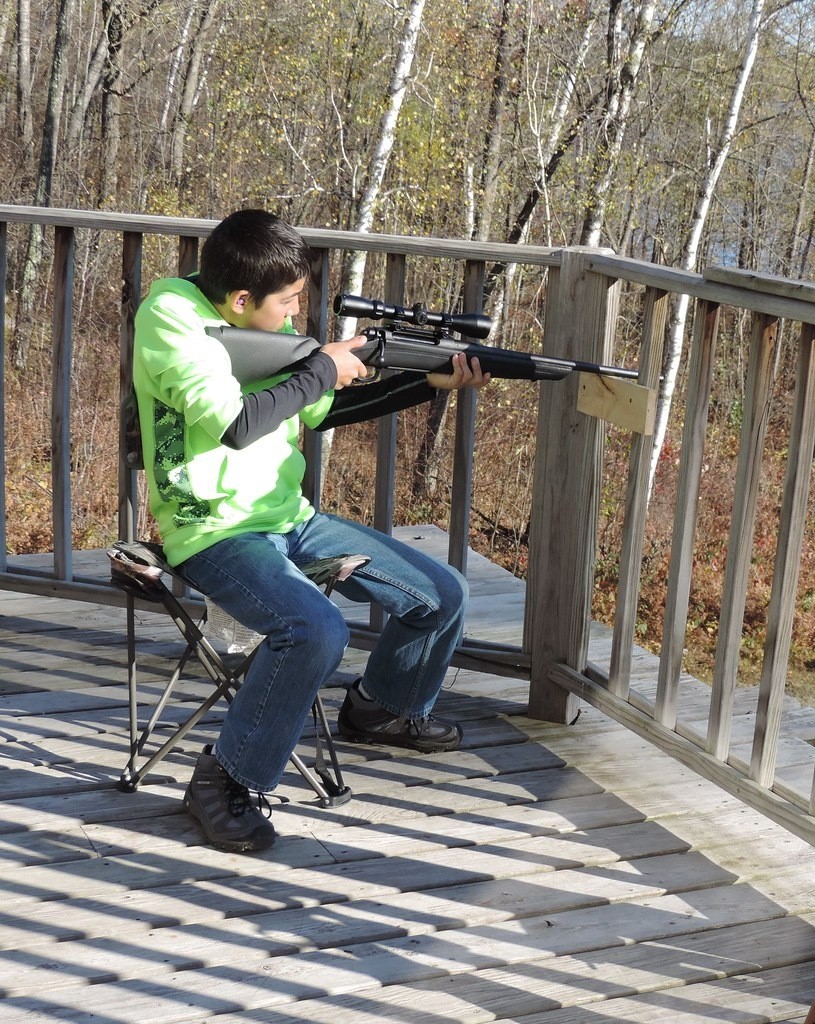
[184,744,275,853]
[337,678,465,753]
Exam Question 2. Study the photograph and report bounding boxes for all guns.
[205,294,638,389]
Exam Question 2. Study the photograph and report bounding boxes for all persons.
[132,207,491,852]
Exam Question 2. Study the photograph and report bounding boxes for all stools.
[106,538,372,807]
